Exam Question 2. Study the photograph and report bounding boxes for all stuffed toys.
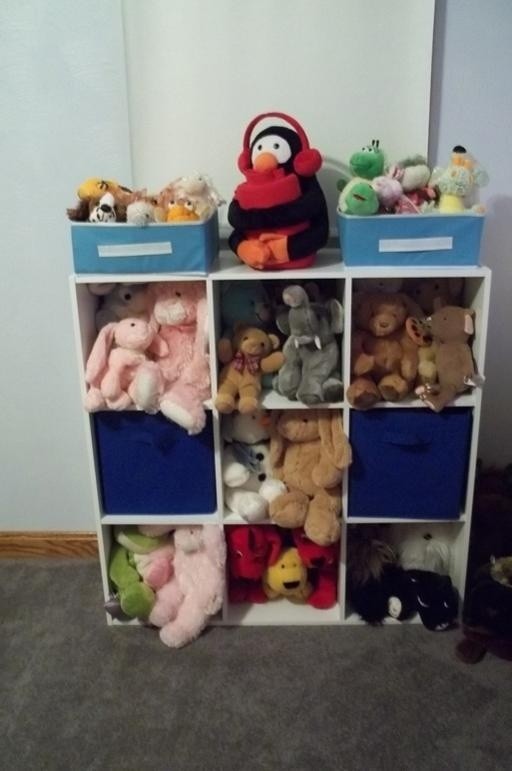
[64,114,512,664]
[64,114,491,649]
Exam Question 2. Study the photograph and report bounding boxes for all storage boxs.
[349,411,472,519]
[95,414,215,513]
[336,204,484,268]
[69,207,220,273]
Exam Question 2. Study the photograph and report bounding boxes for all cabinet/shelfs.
[69,245,493,626]
